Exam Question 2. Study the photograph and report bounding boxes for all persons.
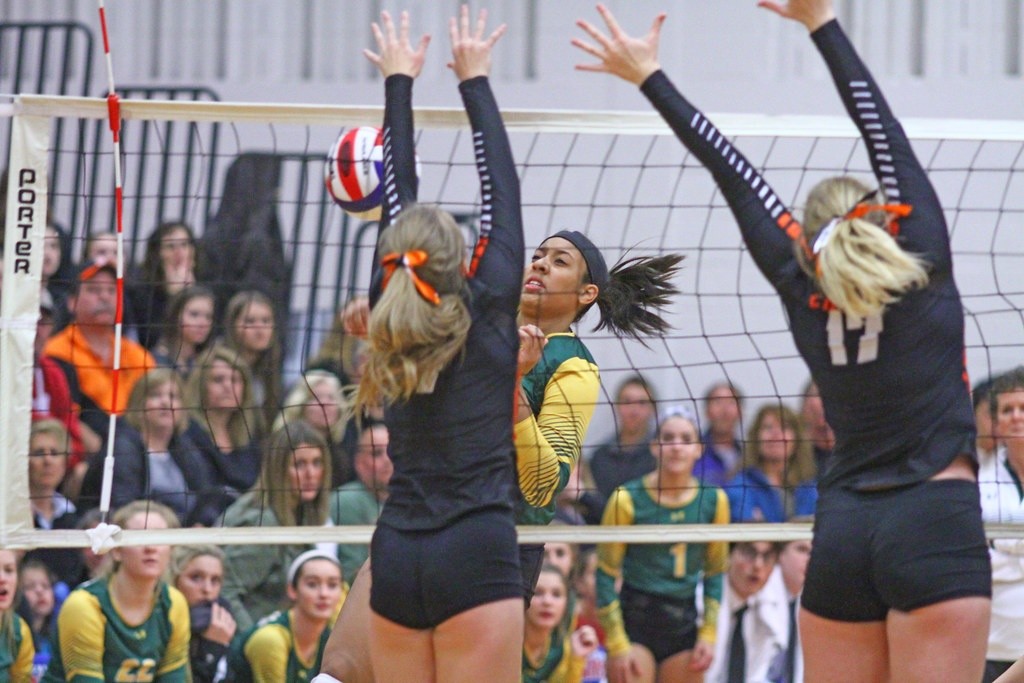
[570,0,994,683]
[0,219,1024,683]
[306,230,691,683]
[363,4,525,683]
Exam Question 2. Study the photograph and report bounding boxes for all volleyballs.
[324,125,421,221]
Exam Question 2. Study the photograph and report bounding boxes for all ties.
[786,599,798,683]
[728,604,748,683]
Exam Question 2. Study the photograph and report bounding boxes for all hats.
[68,255,116,285]
